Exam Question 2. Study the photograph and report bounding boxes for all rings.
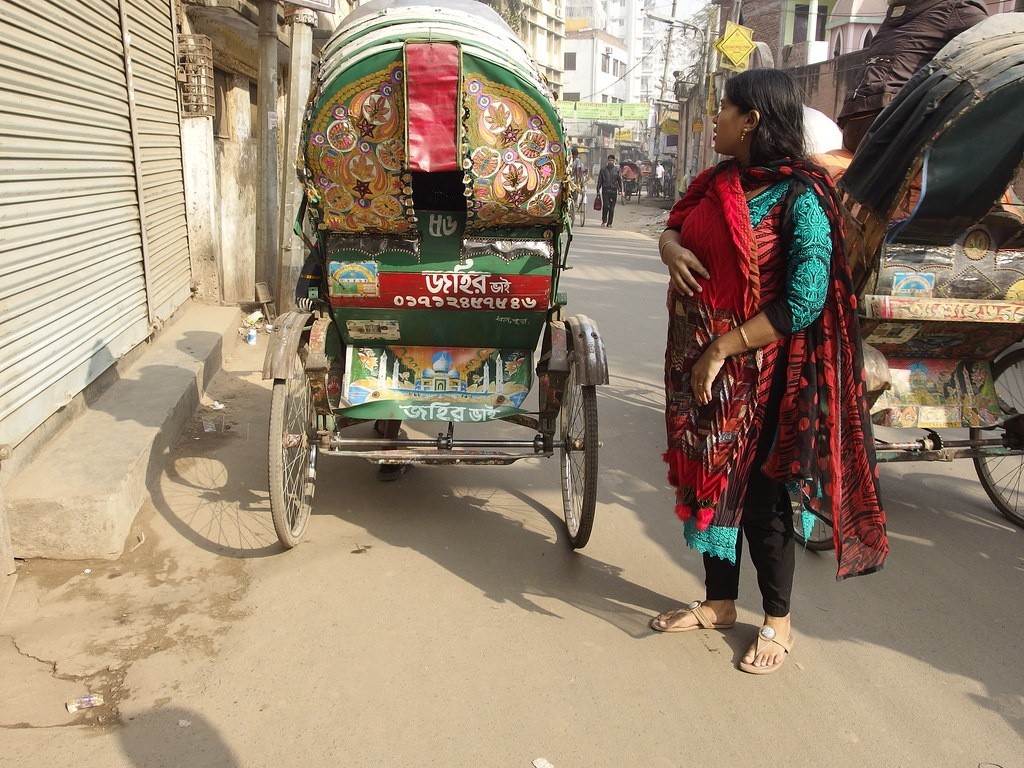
[697,382,704,389]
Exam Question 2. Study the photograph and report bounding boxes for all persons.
[570,145,584,204]
[678,168,693,198]
[636,159,645,179]
[646,65,849,676]
[664,168,672,197]
[837,1,990,154]
[656,159,665,198]
[596,154,622,228]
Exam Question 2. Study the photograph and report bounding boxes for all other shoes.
[601,223,612,228]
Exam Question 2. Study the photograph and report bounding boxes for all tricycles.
[636,159,676,199]
[618,159,643,204]
[566,161,589,227]
[259,1,612,550]
[785,10,1023,549]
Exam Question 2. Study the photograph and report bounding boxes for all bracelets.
[738,324,753,351]
[661,240,680,262]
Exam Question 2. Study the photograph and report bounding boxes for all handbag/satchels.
[594,193,602,211]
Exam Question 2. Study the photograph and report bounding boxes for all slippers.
[737,629,791,674]
[373,419,385,437]
[651,601,737,632]
[377,463,409,480]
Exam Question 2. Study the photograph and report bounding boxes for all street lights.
[647,11,712,190]
